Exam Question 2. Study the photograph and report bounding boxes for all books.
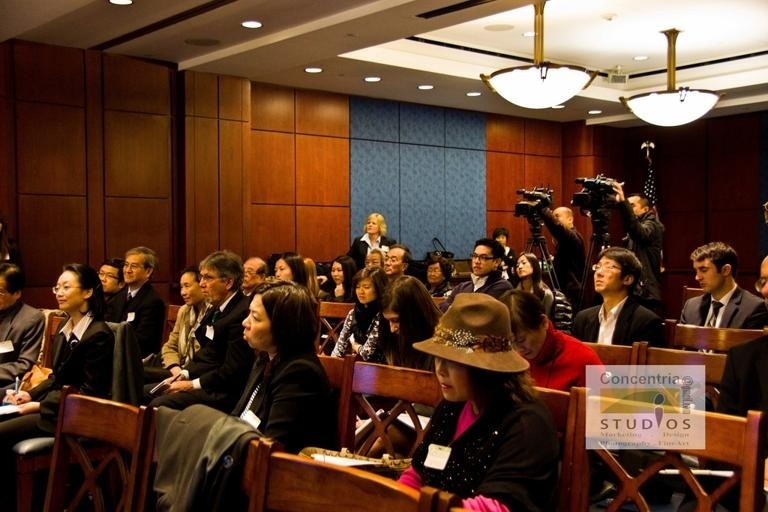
[355,407,432,446]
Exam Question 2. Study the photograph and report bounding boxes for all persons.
[535,205,585,310]
[243,240,557,369]
[503,294,612,494]
[99,260,124,320]
[229,282,342,449]
[571,246,659,347]
[159,267,212,378]
[610,182,663,317]
[0,261,45,392]
[676,241,763,325]
[346,213,396,270]
[394,291,559,512]
[111,247,161,360]
[492,226,518,288]
[0,262,113,494]
[704,254,768,512]
[141,251,250,410]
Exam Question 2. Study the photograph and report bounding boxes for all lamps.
[481,0,598,109]
[621,27,726,127]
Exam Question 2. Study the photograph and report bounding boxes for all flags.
[645,163,666,275]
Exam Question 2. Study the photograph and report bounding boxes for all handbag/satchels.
[18,365,53,393]
[426,238,454,263]
[299,448,412,480]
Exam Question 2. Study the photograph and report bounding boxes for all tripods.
[524,236,561,292]
[572,233,613,322]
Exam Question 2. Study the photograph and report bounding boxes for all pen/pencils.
[15,376,19,394]
[170,373,181,384]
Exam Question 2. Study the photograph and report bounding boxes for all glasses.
[53,285,81,293]
[98,270,119,280]
[593,264,621,271]
[470,253,493,260]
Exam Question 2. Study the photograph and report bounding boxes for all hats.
[412,293,529,373]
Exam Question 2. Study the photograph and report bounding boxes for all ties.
[211,310,220,323]
[127,292,132,300]
[707,301,723,328]
